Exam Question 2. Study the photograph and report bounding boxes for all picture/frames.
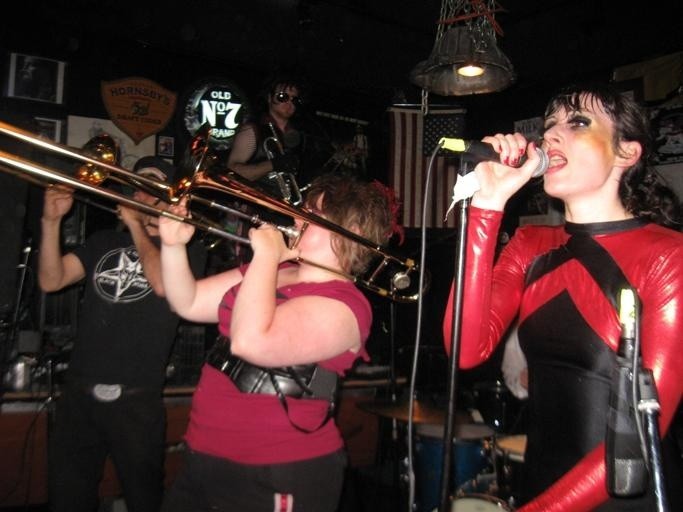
[156,133,176,159]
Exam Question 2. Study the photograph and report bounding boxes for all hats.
[133,156,170,176]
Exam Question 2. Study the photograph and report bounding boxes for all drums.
[399,422,498,511]
[482,433,528,499]
[432,493,517,512]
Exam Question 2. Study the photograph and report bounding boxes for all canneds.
[9,356,31,392]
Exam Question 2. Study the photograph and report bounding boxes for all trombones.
[0,121,433,303]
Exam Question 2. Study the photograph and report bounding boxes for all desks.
[0,378,407,512]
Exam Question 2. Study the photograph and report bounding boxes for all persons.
[32,151,221,510]
[215,66,324,268]
[439,80,683,511]
[151,167,406,512]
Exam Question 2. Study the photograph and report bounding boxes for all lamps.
[413,1,517,117]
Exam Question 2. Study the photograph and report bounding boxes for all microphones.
[441,136,549,178]
[603,293,656,497]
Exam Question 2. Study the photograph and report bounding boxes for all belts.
[68,366,162,403]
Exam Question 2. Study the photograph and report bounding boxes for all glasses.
[275,92,303,106]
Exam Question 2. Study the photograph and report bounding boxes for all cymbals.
[354,397,474,426]
